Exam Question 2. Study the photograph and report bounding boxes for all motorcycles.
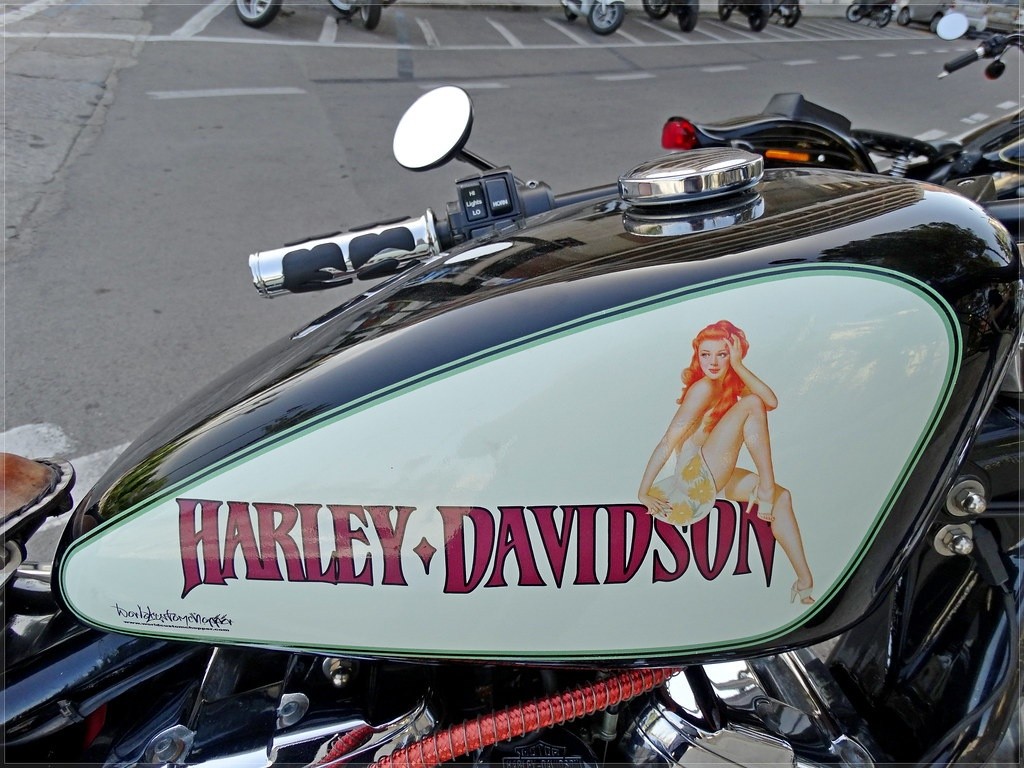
[0,0,1024,768]
